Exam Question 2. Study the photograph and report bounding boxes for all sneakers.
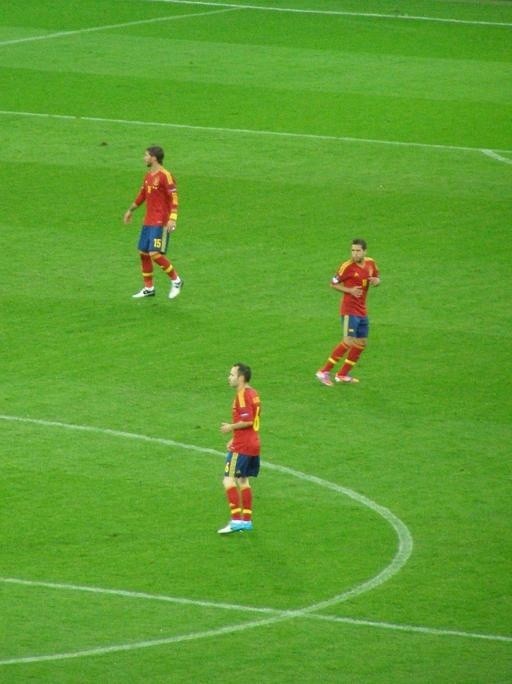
[335,374,360,385]
[217,519,254,535]
[168,276,184,300]
[315,370,335,387]
[132,285,155,298]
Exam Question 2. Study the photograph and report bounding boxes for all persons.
[316,238,380,387]
[124,147,187,298]
[219,362,261,534]
